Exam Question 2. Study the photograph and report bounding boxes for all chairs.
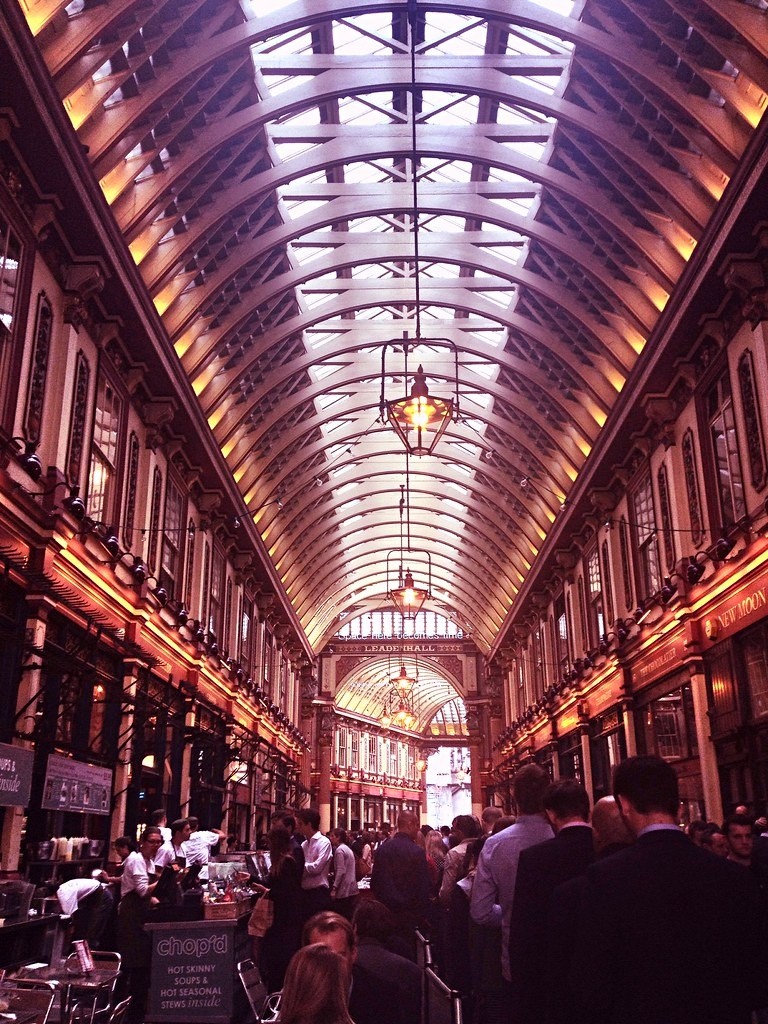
[0,950,131,1024]
[0,880,36,920]
[236,958,283,1024]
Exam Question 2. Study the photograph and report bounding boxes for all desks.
[9,967,122,1024]
[0,914,60,969]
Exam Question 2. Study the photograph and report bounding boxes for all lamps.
[380,688,415,728]
[377,0,461,458]
[389,484,416,701]
[386,328,431,620]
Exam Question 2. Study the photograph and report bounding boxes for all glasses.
[145,838,162,845]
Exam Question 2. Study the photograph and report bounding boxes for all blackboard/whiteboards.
[142,918,238,1024]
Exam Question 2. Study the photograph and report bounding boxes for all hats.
[186,816,199,828]
[151,809,166,821]
[170,819,189,829]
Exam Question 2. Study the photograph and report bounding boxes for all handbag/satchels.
[353,851,371,882]
[247,889,274,937]
[451,843,477,922]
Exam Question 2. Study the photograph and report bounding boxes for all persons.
[572,754,767,1024]
[38,762,768,1023]
